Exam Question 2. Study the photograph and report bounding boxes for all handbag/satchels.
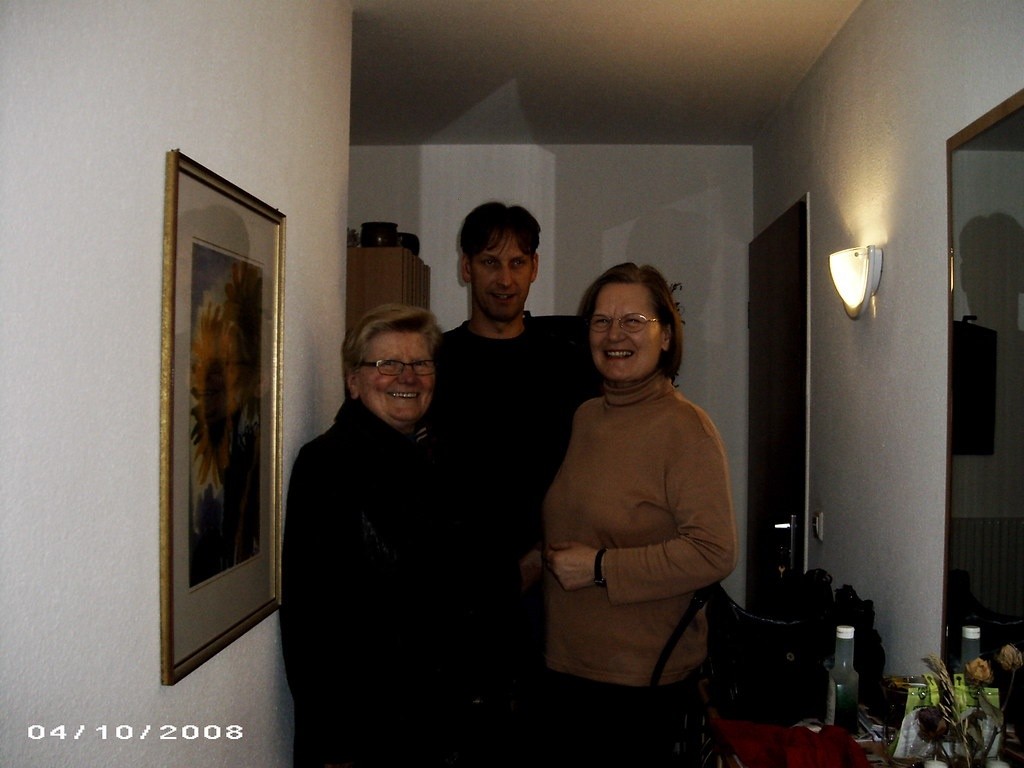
[694,576,889,727]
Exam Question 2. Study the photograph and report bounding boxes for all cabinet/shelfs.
[346,247,432,332]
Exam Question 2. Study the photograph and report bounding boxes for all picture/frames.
[159,150,281,688]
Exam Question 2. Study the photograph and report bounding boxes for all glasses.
[588,311,659,335]
[360,357,437,376]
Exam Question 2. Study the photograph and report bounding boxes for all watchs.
[594,548,607,590]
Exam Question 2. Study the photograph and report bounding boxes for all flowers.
[913,643,1024,768]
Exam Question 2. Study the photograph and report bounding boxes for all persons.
[280,304,498,768]
[432,202,614,768]
[522,261,737,768]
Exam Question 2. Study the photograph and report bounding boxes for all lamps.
[829,245,883,319]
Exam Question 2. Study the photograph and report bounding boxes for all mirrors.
[940,88,1024,768]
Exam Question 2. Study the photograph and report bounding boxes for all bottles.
[826,624,860,735]
[953,624,980,675]
[361,221,421,256]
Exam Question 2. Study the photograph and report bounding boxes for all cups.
[951,675,1004,758]
[882,675,937,765]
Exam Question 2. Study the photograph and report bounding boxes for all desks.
[699,680,905,768]
